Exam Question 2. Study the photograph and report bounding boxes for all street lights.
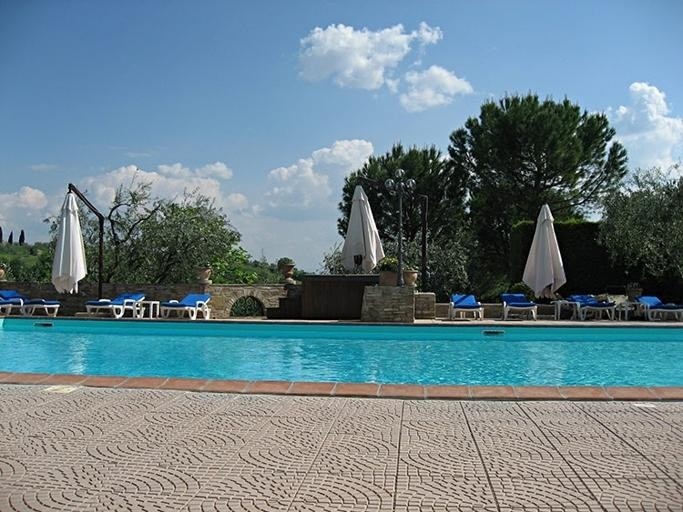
[384,168,417,286]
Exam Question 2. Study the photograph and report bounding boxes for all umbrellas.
[51,191,86,294]
[522,203,566,297]
[341,183,385,274]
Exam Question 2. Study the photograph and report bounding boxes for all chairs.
[0,289,212,323]
[446,291,683,328]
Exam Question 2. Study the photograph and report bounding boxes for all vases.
[401,268,419,286]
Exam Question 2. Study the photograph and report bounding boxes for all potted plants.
[376,256,398,287]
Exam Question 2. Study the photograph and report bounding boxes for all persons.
[1,263,7,280]
